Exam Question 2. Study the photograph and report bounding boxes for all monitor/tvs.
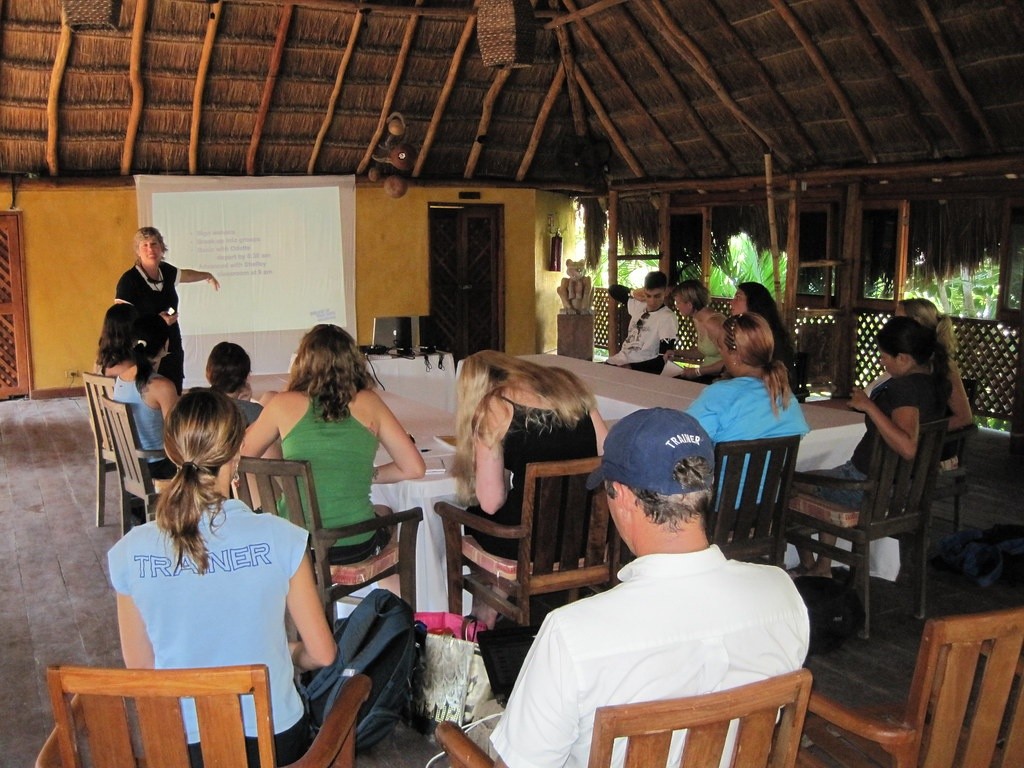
[373,317,411,348]
[419,315,438,353]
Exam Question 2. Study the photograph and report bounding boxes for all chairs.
[35,352,1024,768]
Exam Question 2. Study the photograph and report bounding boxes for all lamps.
[476,0,536,68]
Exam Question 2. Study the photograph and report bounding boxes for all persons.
[785,297,972,582]
[599,270,803,403]
[452,350,609,630]
[489,407,810,768]
[94,302,178,526]
[115,227,220,397]
[206,324,427,599]
[107,387,338,768]
[686,312,810,543]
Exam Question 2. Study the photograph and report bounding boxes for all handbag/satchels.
[414,611,506,759]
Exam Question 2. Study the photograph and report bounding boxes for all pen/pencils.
[419,449,432,453]
[656,353,683,357]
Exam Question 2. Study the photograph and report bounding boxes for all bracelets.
[630,289,634,298]
[694,368,702,376]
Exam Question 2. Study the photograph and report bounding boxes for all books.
[424,456,446,475]
[433,436,458,450]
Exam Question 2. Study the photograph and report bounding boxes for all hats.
[585,407,716,494]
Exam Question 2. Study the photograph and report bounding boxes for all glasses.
[636,312,650,331]
[723,314,742,352]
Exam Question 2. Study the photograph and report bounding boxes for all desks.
[333,419,622,620]
[288,351,456,438]
[456,353,902,582]
[245,373,459,439]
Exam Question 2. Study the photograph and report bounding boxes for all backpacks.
[297,587,418,747]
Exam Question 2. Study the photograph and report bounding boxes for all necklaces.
[141,264,164,292]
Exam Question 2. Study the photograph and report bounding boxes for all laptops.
[477,625,542,708]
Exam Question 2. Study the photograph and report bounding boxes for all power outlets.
[65,370,79,377]
